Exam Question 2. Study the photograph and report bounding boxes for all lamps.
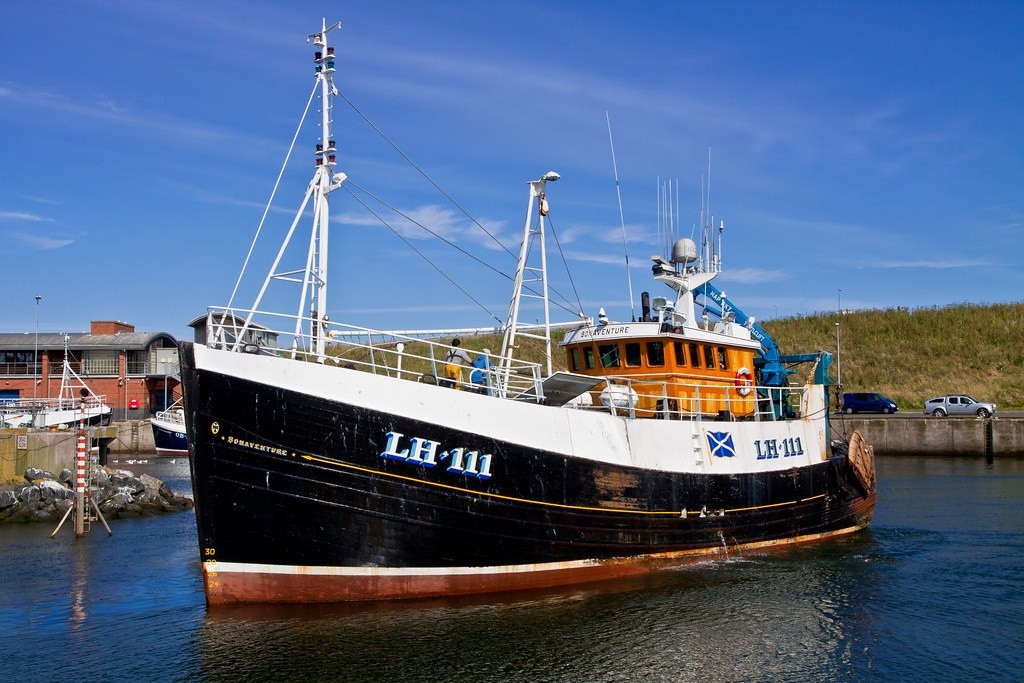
[241,343,260,354]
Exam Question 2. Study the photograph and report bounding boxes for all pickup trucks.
[923,394,997,418]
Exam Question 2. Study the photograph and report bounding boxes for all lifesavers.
[735,367,752,396]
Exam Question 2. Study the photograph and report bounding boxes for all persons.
[446,338,480,391]
[709,352,725,368]
[472,348,494,392]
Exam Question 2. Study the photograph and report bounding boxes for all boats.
[151,404,188,457]
[0,295,112,428]
[177,16,878,607]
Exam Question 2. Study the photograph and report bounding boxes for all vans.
[836,393,898,414]
